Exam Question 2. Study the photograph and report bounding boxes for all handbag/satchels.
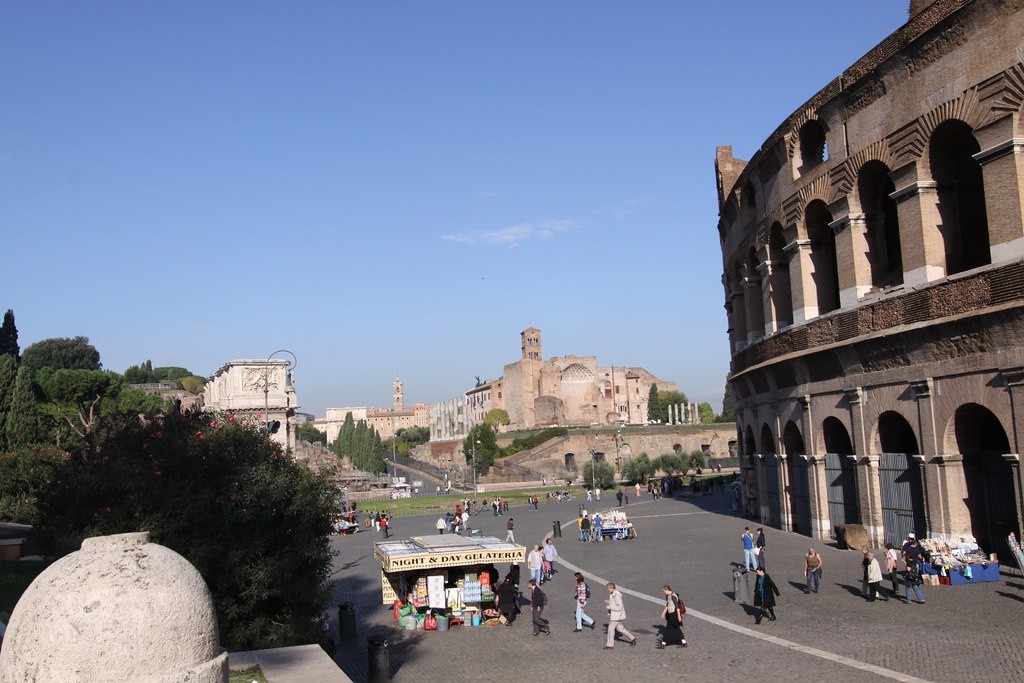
[678,599,686,614]
[585,582,590,598]
[754,545,760,555]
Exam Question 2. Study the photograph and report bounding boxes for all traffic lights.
[267,421,280,433]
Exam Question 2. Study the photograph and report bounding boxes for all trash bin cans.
[552,519,560,540]
[339,603,355,642]
[732,567,750,603]
[579,504,585,517]
[367,633,390,683]
[503,500,509,512]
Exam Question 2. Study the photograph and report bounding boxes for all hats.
[908,533,915,538]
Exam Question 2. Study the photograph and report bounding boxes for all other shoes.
[506,621,511,625]
[805,591,810,594]
[677,643,687,648]
[573,629,582,632]
[886,595,889,601]
[591,620,595,629]
[603,646,612,649]
[545,632,550,636]
[768,616,776,621]
[754,620,761,624]
[813,590,817,593]
[631,638,636,646]
[867,599,874,602]
[658,644,664,649]
[530,632,537,636]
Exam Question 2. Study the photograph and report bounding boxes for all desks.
[593,527,634,540]
[407,597,492,619]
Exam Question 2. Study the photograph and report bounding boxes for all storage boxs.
[921,574,940,585]
[408,570,495,603]
[940,577,950,585]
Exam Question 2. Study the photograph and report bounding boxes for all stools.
[450,618,460,628]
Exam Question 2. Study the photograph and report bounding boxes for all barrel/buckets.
[436,616,448,631]
[472,616,480,626]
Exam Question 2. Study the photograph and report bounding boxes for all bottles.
[463,581,494,601]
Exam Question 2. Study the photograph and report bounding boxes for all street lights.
[393,429,398,484]
[472,428,482,487]
[263,349,297,439]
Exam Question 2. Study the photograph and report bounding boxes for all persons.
[573,572,596,633]
[603,582,637,650]
[616,489,630,507]
[552,475,556,485]
[566,478,572,486]
[527,538,558,589]
[528,579,550,636]
[804,546,822,594]
[477,562,523,626]
[717,464,723,473]
[635,482,641,497]
[655,584,688,649]
[647,475,730,502]
[505,517,516,543]
[711,464,716,474]
[436,504,470,535]
[741,527,766,572]
[731,471,738,482]
[332,500,393,539]
[542,475,546,486]
[578,512,603,543]
[754,567,780,624]
[414,487,419,497]
[696,464,702,475]
[463,487,604,516]
[861,532,930,605]
